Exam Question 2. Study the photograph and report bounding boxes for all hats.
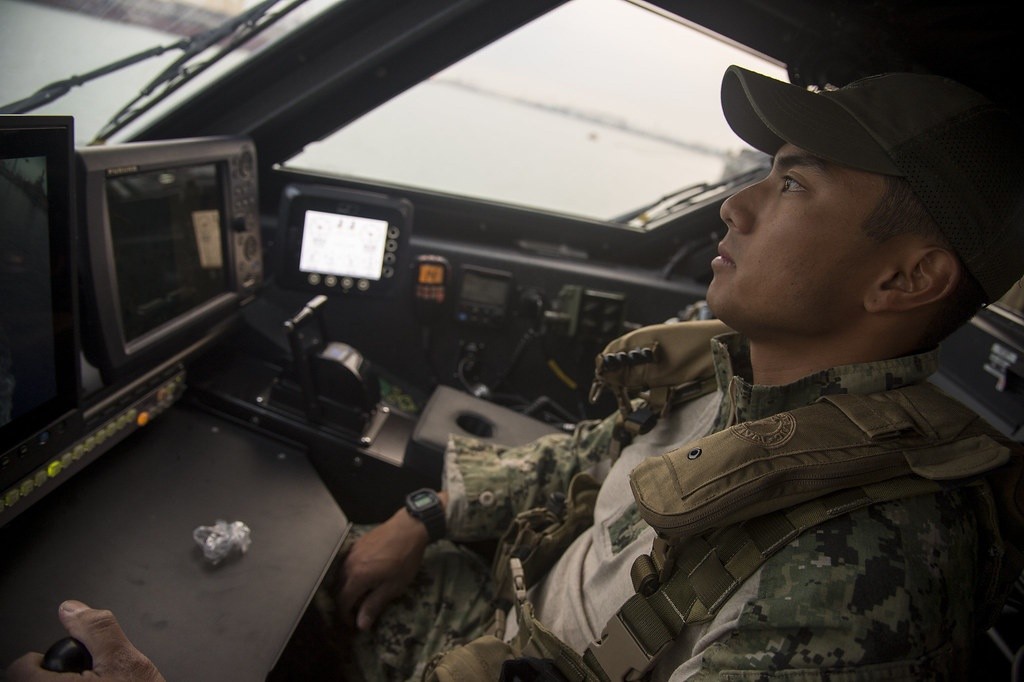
[720,65,1024,304]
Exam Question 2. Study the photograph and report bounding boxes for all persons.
[1,65,1024,682]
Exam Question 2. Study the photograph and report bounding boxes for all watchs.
[405,488,447,546]
